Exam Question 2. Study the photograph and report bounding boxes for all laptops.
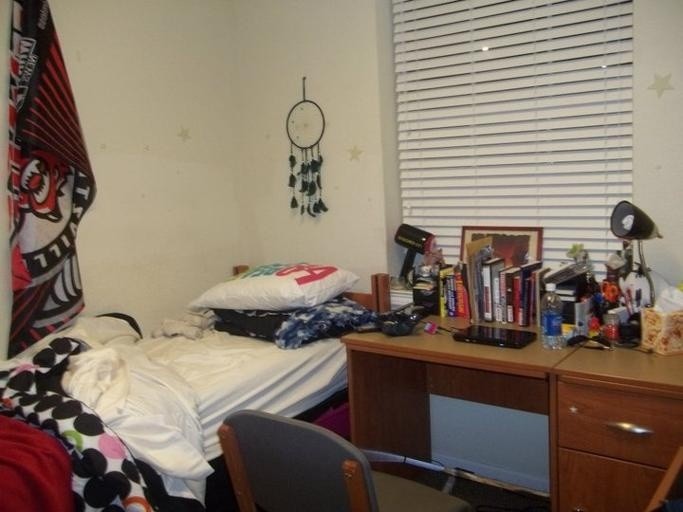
[452,325,537,349]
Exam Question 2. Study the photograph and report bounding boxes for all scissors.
[601,281,619,301]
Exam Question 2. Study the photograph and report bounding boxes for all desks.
[338,305,683,511]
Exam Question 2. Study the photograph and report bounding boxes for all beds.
[1,264,391,512]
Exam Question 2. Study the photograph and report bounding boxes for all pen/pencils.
[625,288,641,316]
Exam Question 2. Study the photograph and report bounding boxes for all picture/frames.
[461,225,543,264]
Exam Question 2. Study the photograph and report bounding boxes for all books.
[438,244,590,330]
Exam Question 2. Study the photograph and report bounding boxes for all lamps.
[611,197,664,305]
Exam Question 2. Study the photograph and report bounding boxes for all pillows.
[184,258,384,351]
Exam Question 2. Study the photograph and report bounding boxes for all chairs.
[218,409,474,511]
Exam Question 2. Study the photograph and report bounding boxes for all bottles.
[603,314,621,344]
[540,282,566,351]
